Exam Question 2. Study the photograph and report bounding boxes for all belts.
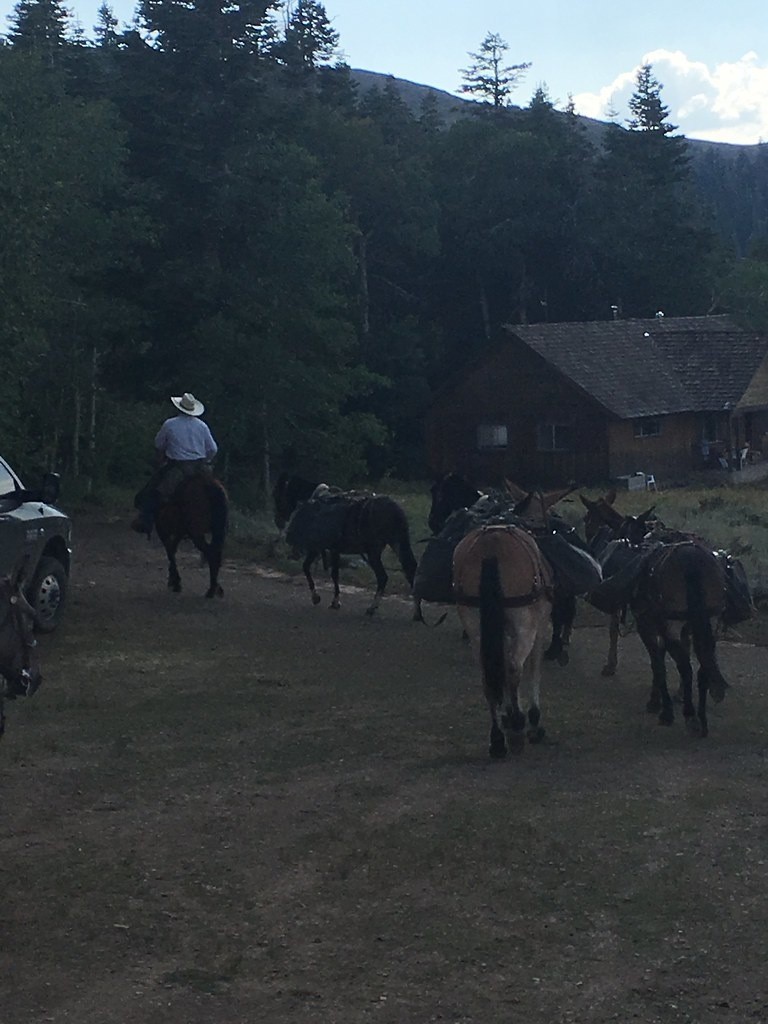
[168,458,208,465]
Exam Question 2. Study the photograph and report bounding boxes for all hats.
[171,393,205,416]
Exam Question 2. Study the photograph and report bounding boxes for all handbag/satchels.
[719,551,753,625]
[413,509,468,602]
[286,499,351,548]
[582,540,650,614]
[535,533,603,594]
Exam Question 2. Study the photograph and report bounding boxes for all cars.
[0,454,75,636]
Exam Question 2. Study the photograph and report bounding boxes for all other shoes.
[131,512,154,533]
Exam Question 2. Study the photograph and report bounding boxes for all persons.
[130,393,218,534]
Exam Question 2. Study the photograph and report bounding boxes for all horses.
[130,464,231,599]
[269,468,731,761]
[0,552,44,742]
